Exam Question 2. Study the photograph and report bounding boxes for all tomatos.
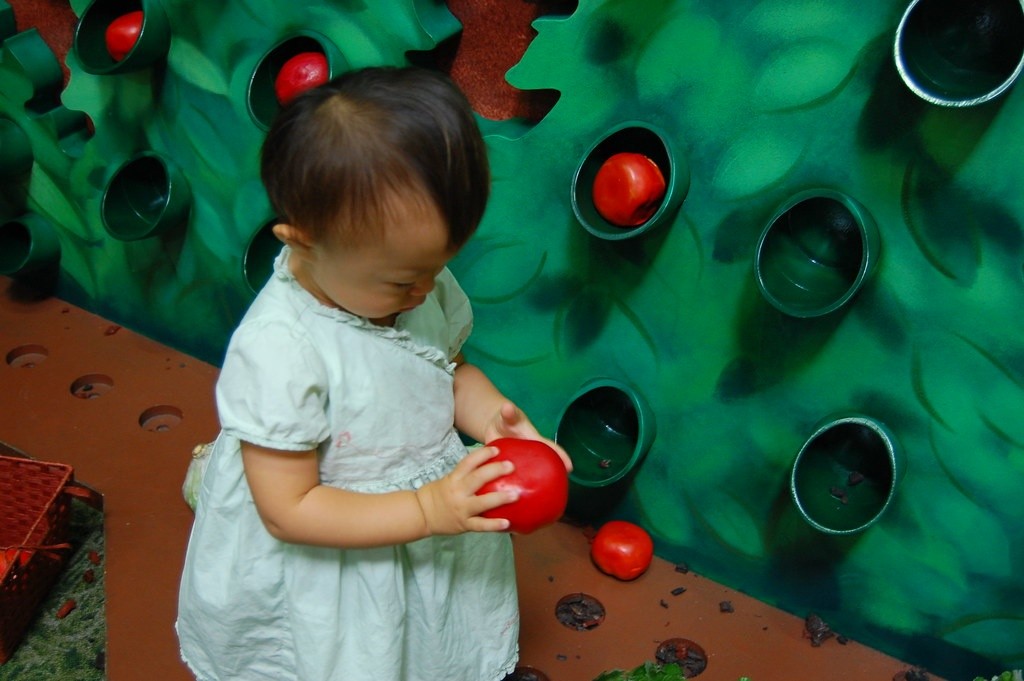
[473,438,567,536]
[105,8,143,62]
[593,152,667,228]
[274,51,327,105]
[591,521,654,580]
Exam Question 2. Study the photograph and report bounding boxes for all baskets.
[0,456,103,665]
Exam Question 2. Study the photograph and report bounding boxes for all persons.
[175,65,573,680]
